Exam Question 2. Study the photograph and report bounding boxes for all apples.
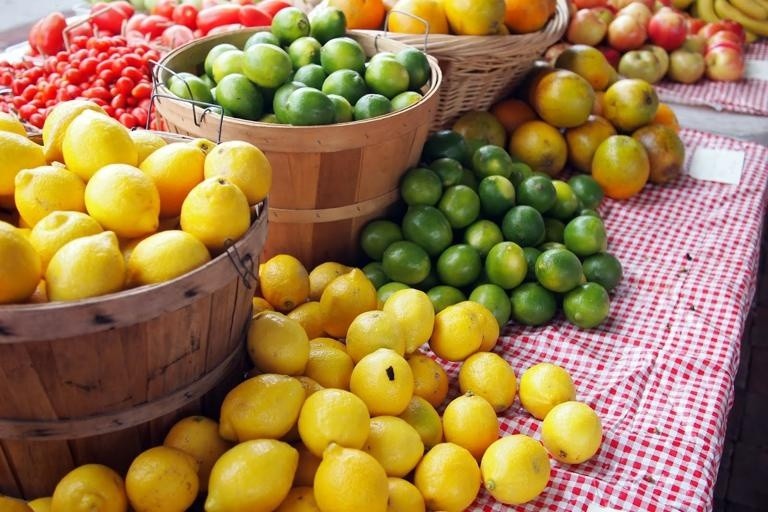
[546,0,746,82]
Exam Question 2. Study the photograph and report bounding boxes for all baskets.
[374,2,568,135]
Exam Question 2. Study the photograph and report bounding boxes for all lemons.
[0,253,603,511]
[0,96,272,302]
[166,0,551,127]
[363,131,622,329]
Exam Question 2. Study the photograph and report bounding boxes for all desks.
[0,1,767,511]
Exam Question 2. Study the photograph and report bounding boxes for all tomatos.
[0,0,293,129]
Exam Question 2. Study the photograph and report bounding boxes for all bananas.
[693,0,768,45]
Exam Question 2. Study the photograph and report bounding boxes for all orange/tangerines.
[459,44,685,201]
